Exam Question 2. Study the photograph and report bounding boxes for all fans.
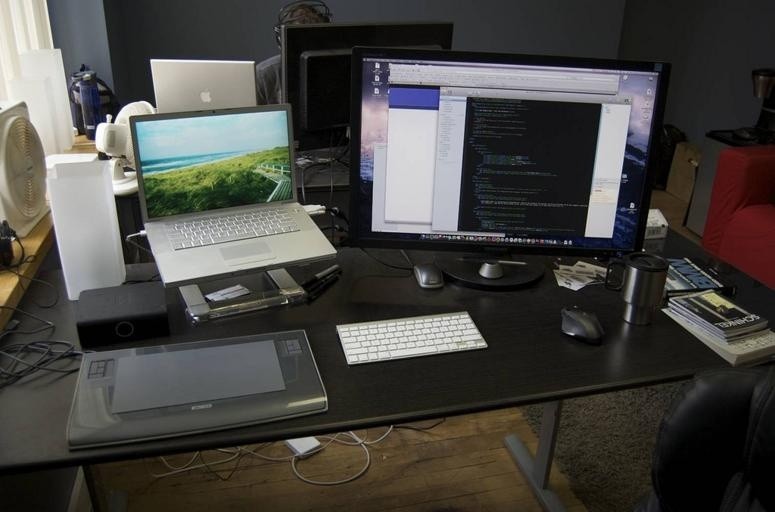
[0,102,51,239]
[94,100,157,197]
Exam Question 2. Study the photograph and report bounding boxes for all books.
[661,288,775,367]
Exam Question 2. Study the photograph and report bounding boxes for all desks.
[0,222,775,512]
[99,149,354,262]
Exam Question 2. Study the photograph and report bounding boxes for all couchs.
[703,144,775,290]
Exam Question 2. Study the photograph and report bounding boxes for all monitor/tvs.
[149,57,258,113]
[347,45,672,289]
[280,20,455,151]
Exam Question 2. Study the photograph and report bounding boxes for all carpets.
[519,379,690,512]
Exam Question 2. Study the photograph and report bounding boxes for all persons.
[255,3,350,152]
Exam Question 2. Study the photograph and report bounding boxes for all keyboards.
[335,310,488,365]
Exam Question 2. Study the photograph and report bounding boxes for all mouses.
[414,262,445,289]
[559,305,605,345]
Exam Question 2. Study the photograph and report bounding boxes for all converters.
[286,435,321,458]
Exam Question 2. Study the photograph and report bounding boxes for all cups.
[604,252,669,326]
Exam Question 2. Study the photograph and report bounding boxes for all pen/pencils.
[308,269,343,294]
[301,264,339,292]
[304,275,340,305]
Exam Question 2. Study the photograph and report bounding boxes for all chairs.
[647,366,774,512]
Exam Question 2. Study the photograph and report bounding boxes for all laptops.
[126,102,338,288]
[65,327,330,450]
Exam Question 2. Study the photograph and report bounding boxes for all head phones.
[271,0,331,51]
